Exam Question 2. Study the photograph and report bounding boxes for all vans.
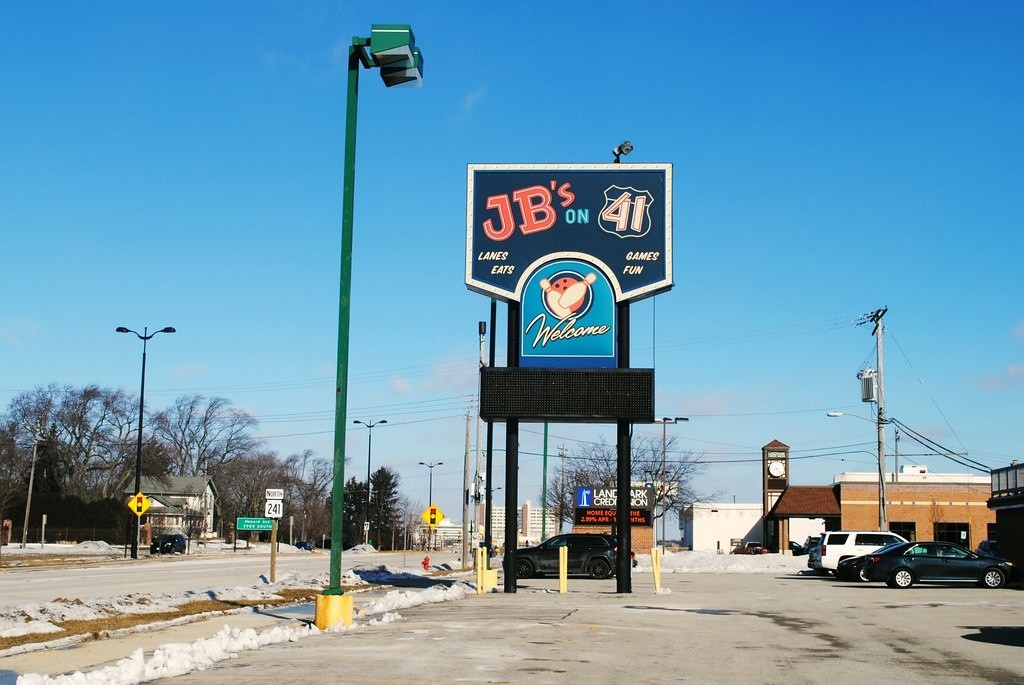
[804,536,821,552]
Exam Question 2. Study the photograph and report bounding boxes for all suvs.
[150,534,186,555]
[745,542,763,554]
[502,533,618,579]
[816,530,912,575]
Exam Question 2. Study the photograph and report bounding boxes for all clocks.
[769,461,784,476]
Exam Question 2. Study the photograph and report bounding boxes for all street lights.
[490,487,502,550]
[116,327,176,560]
[655,417,689,552]
[827,412,887,530]
[352,419,388,544]
[644,469,671,547]
[419,461,443,550]
[614,141,633,592]
[323,22,427,594]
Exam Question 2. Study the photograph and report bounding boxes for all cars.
[837,542,966,582]
[808,547,820,572]
[863,542,1016,589]
[297,541,313,551]
[788,541,803,556]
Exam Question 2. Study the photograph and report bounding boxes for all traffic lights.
[429,509,437,523]
[137,497,142,513]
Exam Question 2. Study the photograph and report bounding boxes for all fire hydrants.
[421,557,429,570]
